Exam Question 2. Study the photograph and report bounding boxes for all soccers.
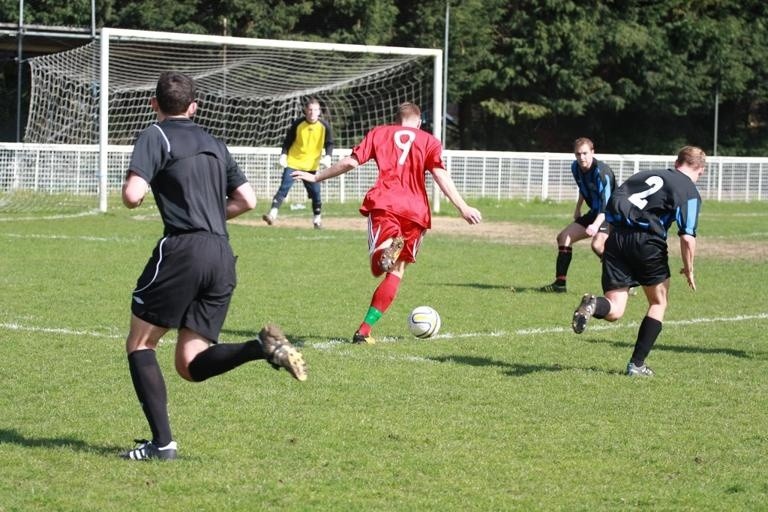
[408,305,441,338]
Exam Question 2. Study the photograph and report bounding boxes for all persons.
[262,99,333,229]
[120,70,306,462]
[541,138,635,294]
[572,147,706,376]
[289,102,482,344]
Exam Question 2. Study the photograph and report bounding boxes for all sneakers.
[624,362,657,377]
[261,214,274,226]
[314,222,325,230]
[351,330,376,346]
[572,291,596,334]
[377,235,406,272]
[117,435,180,462]
[540,282,568,294]
[258,322,309,383]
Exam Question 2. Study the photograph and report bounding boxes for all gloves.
[319,155,333,168]
[279,154,288,168]
